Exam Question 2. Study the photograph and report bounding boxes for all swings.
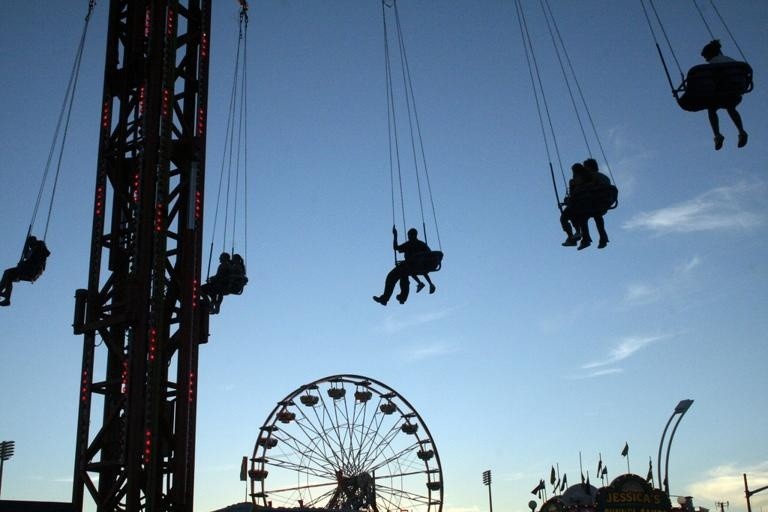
[382,0,443,274]
[16,1,95,281]
[206,1,248,294]
[515,1,620,219]
[639,0,753,110]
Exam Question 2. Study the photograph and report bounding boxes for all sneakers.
[416,281,425,293]
[597,237,610,249]
[714,134,725,150]
[373,295,388,306]
[396,294,404,304]
[0,289,11,306]
[561,233,591,250]
[737,131,748,148]
[429,284,436,294]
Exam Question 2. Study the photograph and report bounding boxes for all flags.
[239,455,248,481]
[530,442,654,499]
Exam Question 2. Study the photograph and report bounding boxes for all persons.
[371,224,419,306]
[208,254,248,314]
[0,235,40,292]
[559,163,588,248]
[403,249,438,295]
[199,251,231,307]
[563,157,613,251]
[0,241,51,306]
[701,40,749,151]
